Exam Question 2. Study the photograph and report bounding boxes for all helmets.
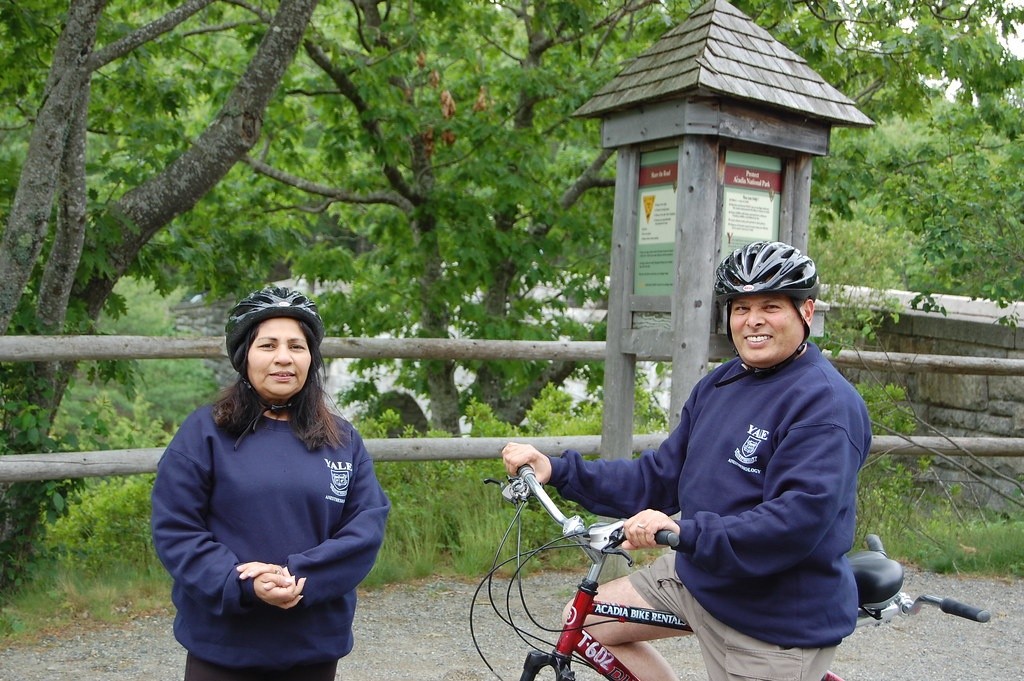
[714,241,820,308]
[225,286,324,370]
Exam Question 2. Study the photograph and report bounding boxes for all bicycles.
[469,465,991,680]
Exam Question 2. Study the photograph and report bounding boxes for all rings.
[275,571,281,575]
[638,523,644,528]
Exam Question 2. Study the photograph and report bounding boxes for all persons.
[150,287,390,681]
[501,241,872,681]
